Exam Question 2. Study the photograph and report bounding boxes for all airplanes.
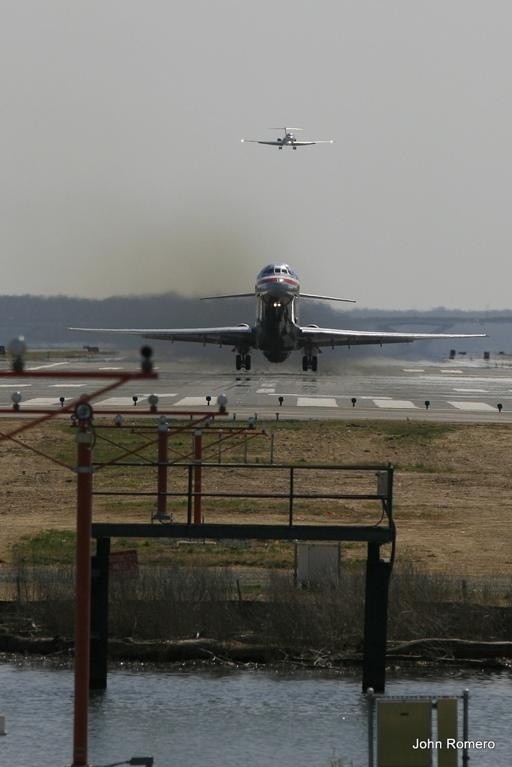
[69,261,489,371]
[240,126,334,151]
